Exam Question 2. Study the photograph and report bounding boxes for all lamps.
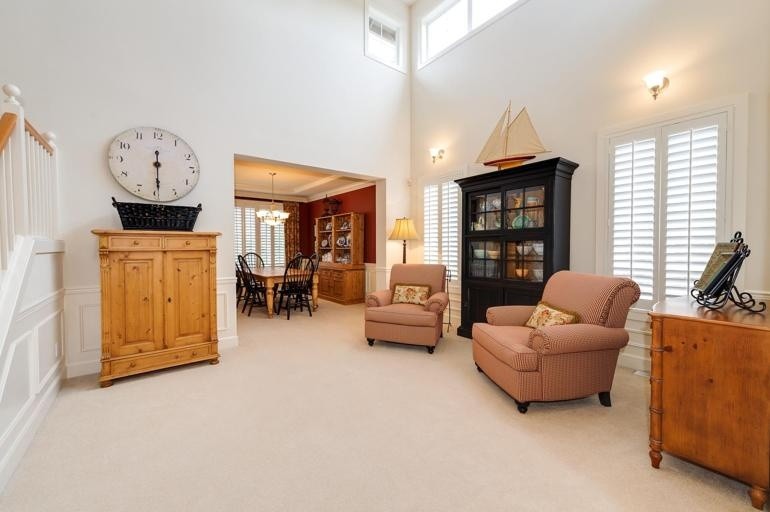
[256,172,291,227]
[387,215,421,263]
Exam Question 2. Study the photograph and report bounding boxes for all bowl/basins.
[515,243,545,282]
[527,197,539,206]
[473,248,502,258]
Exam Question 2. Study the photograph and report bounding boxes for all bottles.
[478,217,484,230]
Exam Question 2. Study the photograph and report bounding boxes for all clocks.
[107,125,201,203]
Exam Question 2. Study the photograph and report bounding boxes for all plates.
[493,199,501,209]
[337,236,345,246]
[512,215,530,229]
[321,240,328,247]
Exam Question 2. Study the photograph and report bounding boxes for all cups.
[513,198,522,208]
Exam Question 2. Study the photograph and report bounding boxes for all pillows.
[390,282,432,307]
[521,299,580,330]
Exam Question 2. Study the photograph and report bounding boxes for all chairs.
[364,263,450,353]
[235,251,322,320]
[471,266,642,413]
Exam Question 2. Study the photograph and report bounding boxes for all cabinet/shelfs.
[91,227,222,388]
[315,212,367,306]
[646,294,770,508]
[453,156,580,340]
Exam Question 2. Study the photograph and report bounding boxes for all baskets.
[111,197,202,231]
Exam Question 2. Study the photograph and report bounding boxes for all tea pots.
[480,201,494,211]
